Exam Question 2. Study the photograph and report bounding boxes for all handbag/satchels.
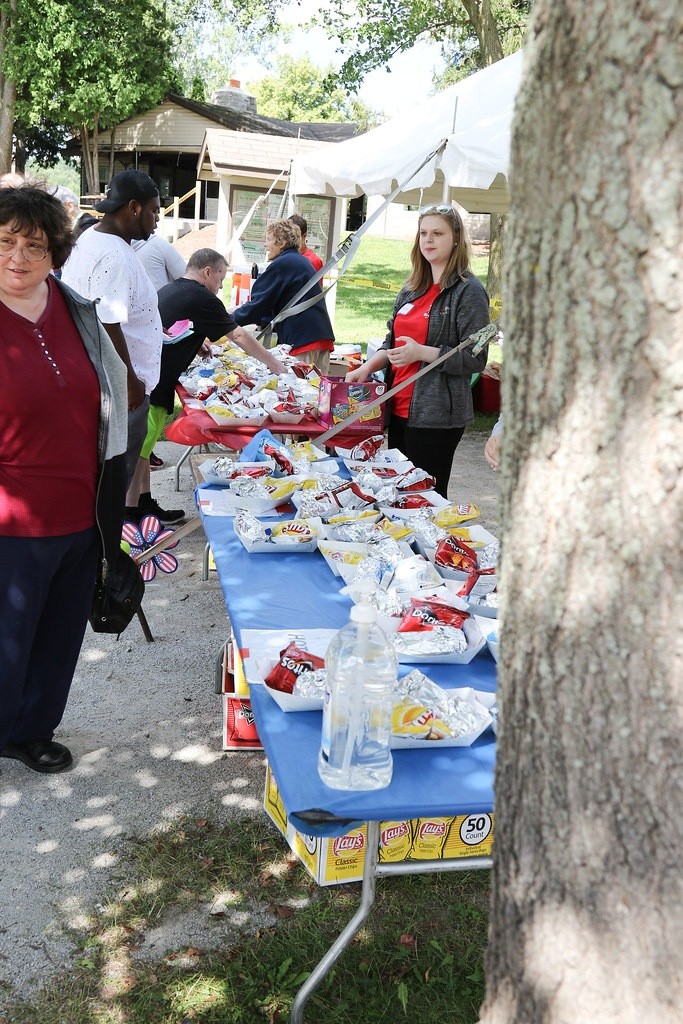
[87,549,145,641]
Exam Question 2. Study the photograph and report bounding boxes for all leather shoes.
[0,739,73,772]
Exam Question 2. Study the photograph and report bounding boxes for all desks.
[188,449,502,1024]
[163,342,385,492]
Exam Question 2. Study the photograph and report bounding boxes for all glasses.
[0,236,51,262]
[422,204,454,218]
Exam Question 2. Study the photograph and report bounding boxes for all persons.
[0,184,128,773]
[344,204,491,501]
[0,172,335,542]
[483,413,503,471]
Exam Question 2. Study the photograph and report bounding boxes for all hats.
[93,170,160,213]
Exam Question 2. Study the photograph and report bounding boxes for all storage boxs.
[328,360,350,377]
[367,338,386,373]
[222,641,266,750]
[316,375,387,436]
[330,344,362,361]
[262,759,496,888]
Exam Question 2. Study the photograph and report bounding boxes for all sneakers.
[147,498,186,524]
[149,451,164,469]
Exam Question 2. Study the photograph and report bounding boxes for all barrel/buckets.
[472,372,500,413]
[367,337,387,370]
[231,265,252,306]
[330,344,362,372]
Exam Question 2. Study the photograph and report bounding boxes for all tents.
[286,47,524,214]
